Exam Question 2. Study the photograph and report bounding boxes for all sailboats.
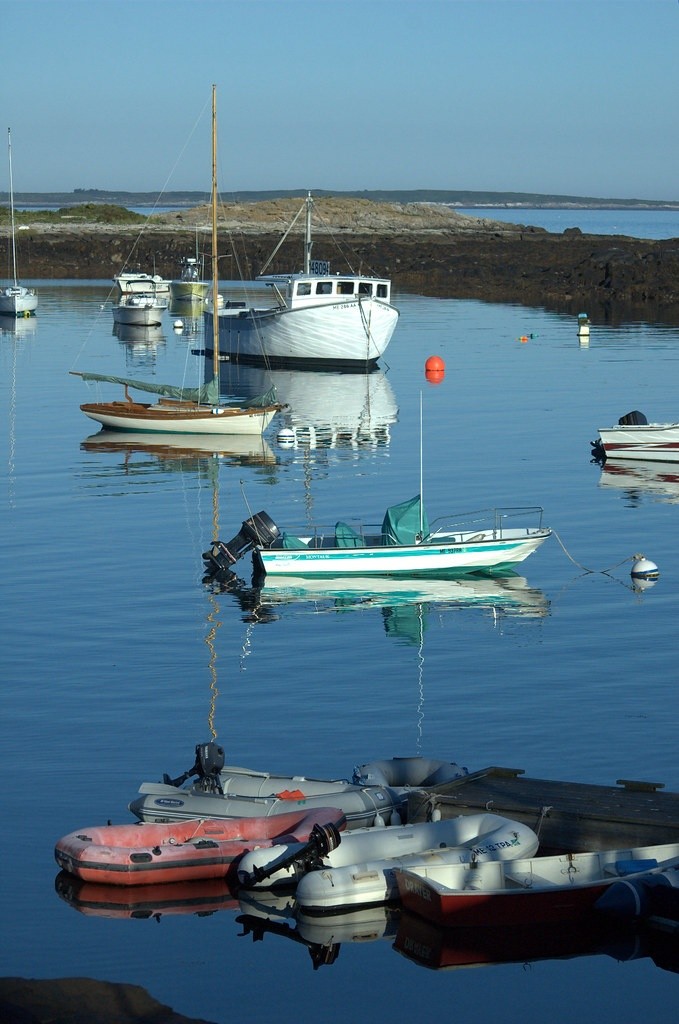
[0,126,38,313]
[60,81,296,434]
[0,311,38,505]
[74,425,278,736]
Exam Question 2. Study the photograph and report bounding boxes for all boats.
[231,903,390,947]
[111,320,168,371]
[199,186,400,368]
[252,568,552,747]
[598,463,679,501]
[352,755,470,810]
[392,912,671,969]
[167,299,208,320]
[111,275,167,326]
[53,806,347,884]
[232,812,547,914]
[586,410,679,466]
[168,256,210,302]
[394,842,679,929]
[201,390,554,580]
[202,360,398,464]
[55,867,239,920]
[126,737,399,834]
